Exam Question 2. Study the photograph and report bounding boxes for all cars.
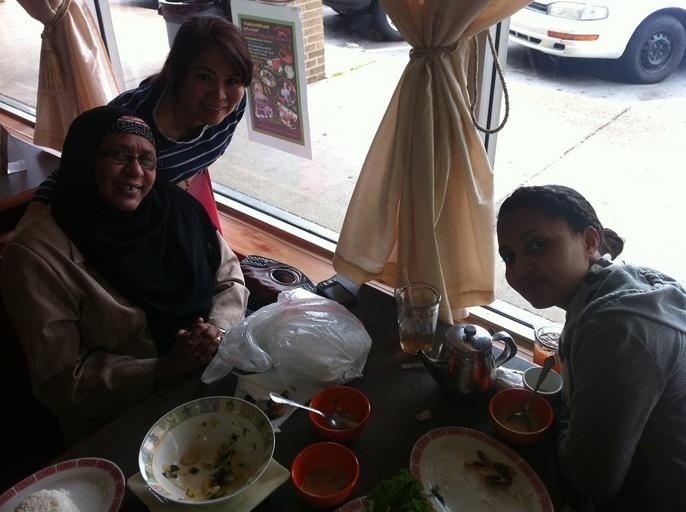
[507,2,686,83]
[322,0,406,45]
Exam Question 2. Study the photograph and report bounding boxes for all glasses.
[97,147,160,170]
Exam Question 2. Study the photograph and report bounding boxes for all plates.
[410,427,554,511]
[0,457,126,512]
[127,459,291,512]
[251,47,299,130]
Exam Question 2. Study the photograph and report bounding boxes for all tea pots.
[416,323,517,399]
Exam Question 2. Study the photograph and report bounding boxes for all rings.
[214,336,221,344]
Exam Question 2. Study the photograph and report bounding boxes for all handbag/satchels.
[240,254,317,312]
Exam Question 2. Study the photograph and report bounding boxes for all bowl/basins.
[291,387,370,506]
[489,367,563,437]
[137,395,276,505]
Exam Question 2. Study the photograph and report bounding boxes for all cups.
[534,325,563,374]
[394,282,442,356]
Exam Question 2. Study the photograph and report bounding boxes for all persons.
[494,183,686,512]
[0,104,249,425]
[106,15,254,238]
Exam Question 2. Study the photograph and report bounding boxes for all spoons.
[270,392,346,430]
[508,356,555,430]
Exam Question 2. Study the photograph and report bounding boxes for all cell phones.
[317,278,358,311]
